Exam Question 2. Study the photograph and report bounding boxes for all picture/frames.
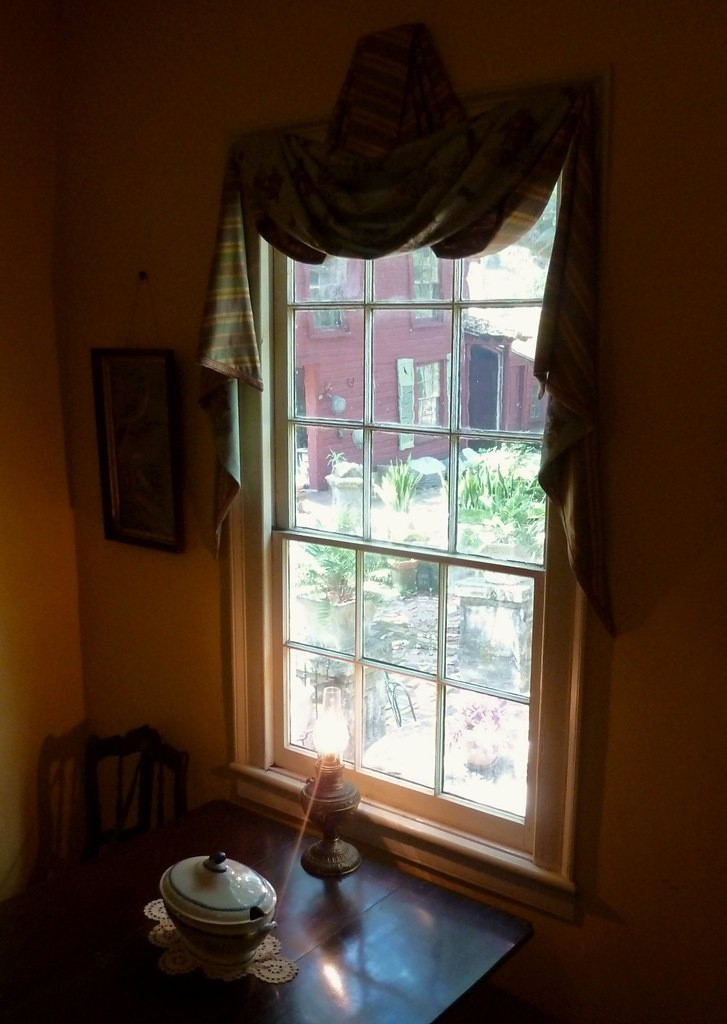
[90,346,182,553]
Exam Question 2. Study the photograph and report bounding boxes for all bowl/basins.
[159,851,279,972]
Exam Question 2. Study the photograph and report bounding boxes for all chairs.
[85,724,190,855]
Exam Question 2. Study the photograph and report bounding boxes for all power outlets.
[184,748,204,776]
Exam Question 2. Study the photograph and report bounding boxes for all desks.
[0,798,533,1024]
